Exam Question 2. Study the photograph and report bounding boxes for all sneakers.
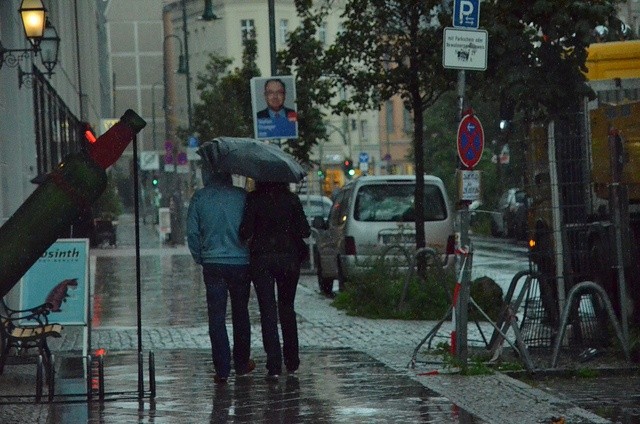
[213,374,228,383]
[235,359,256,375]
[265,372,280,381]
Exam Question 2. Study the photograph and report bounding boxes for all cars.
[297,196,333,244]
[491,188,532,240]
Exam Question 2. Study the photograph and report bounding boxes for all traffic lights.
[343,160,353,178]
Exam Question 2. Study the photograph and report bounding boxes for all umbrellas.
[196,136,308,195]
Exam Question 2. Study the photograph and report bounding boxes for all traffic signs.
[442,27,489,71]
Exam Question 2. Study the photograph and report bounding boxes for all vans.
[310,175,456,295]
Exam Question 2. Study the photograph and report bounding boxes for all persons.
[186,171,256,382]
[257,79,296,135]
[238,182,312,380]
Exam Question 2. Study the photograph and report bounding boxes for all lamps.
[18,20,61,89]
[0,1,47,70]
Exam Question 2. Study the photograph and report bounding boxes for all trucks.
[517,41,640,327]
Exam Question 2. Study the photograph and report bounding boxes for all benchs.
[0,296,64,402]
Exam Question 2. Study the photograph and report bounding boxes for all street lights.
[162,33,185,141]
[181,0,223,190]
[151,81,166,149]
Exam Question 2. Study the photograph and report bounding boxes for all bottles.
[0,109,147,298]
